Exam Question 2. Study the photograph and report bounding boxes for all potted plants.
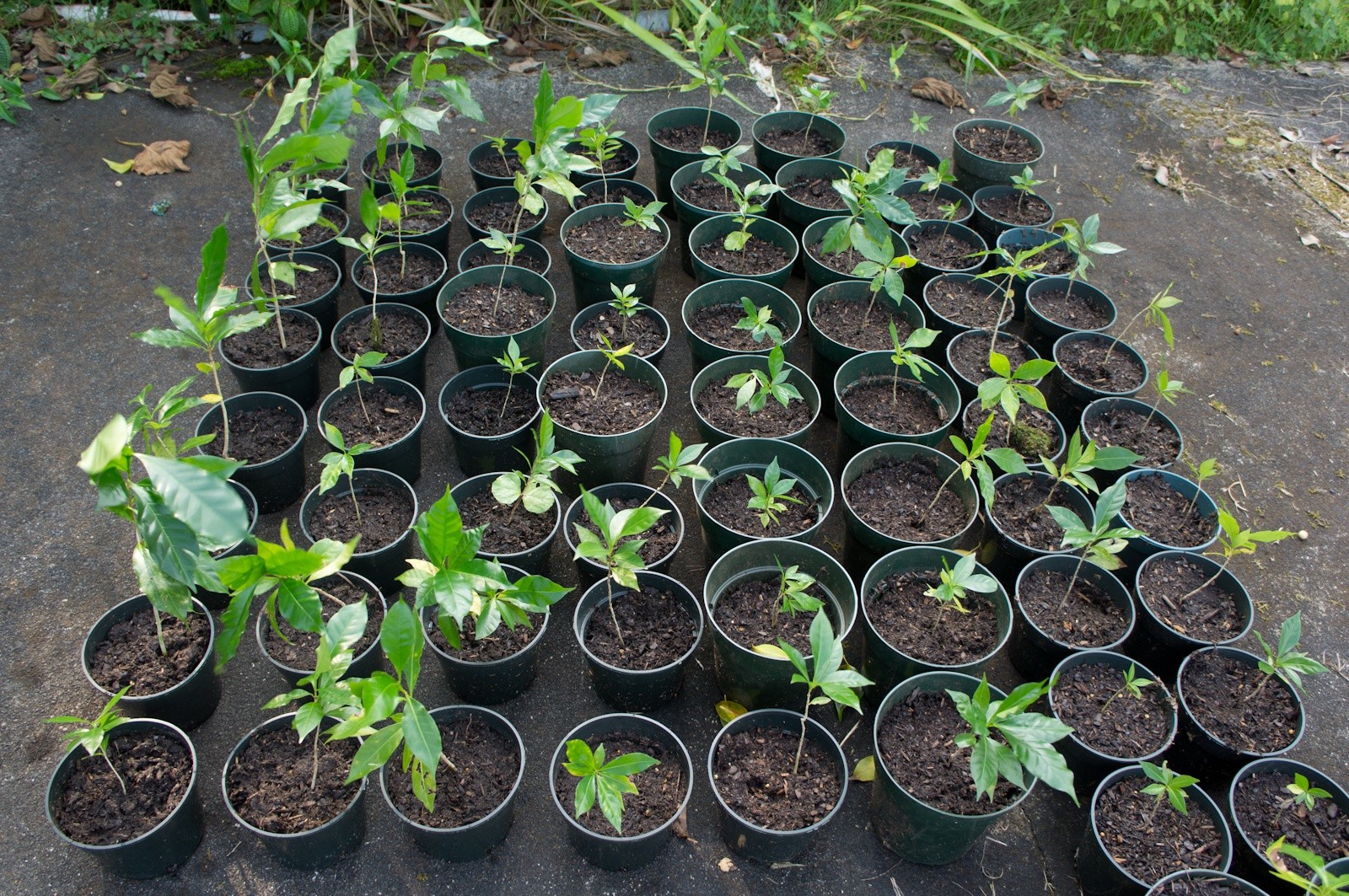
[41,94,1349,896]
